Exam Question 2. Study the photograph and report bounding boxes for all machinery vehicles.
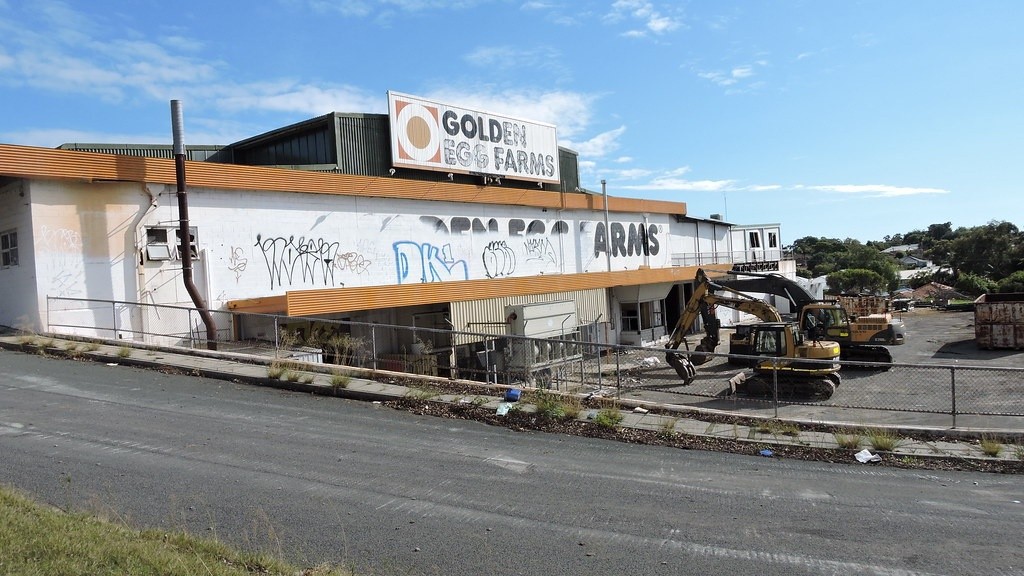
[667,282,840,400]
[691,265,907,370]
